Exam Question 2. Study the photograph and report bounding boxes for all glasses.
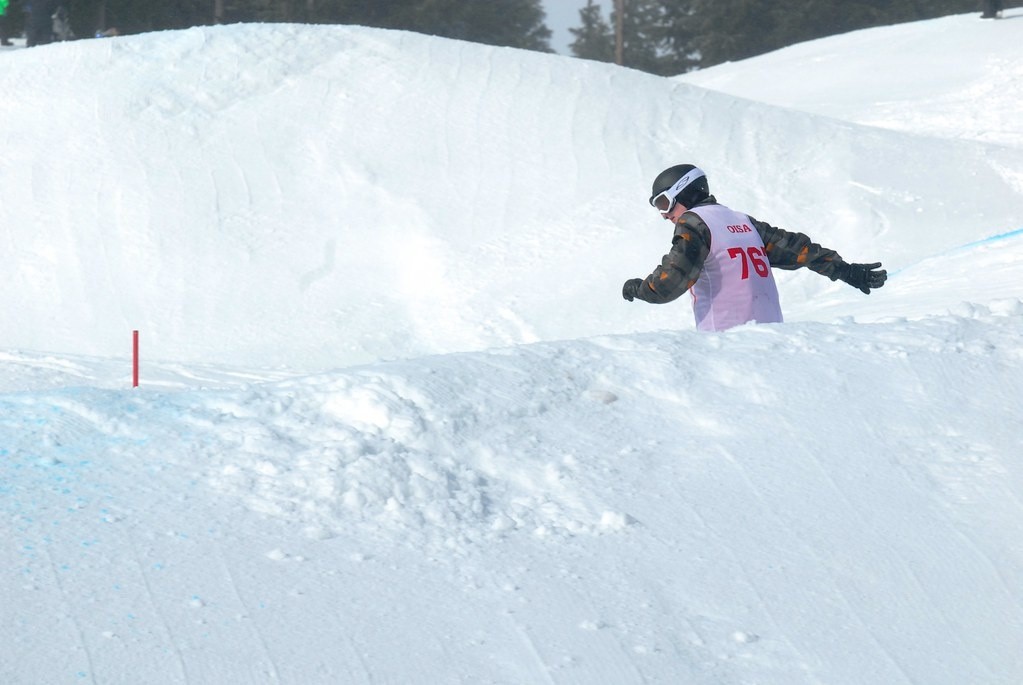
[652,191,676,214]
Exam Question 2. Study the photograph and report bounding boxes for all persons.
[622,164,887,332]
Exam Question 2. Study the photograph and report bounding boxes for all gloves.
[623,278,643,302]
[840,262,887,294]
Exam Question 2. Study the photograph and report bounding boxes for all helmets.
[649,164,708,211]
